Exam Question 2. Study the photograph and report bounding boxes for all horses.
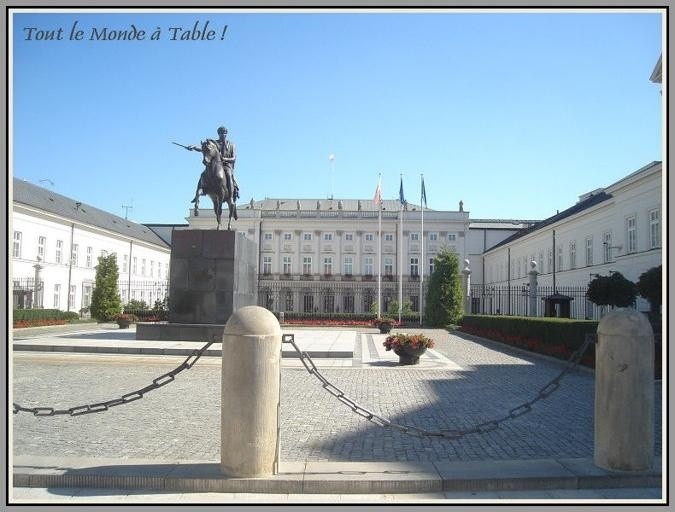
[193,138,236,232]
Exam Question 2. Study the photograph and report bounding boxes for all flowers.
[373,317,400,327]
[113,313,134,320]
[383,333,435,355]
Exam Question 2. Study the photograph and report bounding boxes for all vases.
[116,321,131,330]
[380,327,391,334]
[398,355,420,366]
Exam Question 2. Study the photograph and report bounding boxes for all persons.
[186,126,241,204]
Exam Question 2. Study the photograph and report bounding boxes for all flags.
[372,185,381,206]
[420,178,429,209]
[398,178,407,205]
